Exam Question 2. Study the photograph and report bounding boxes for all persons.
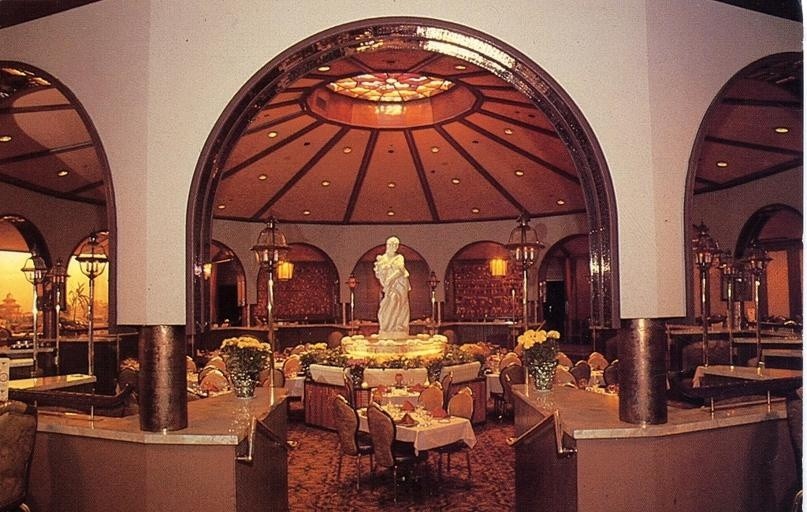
[373,236,411,332]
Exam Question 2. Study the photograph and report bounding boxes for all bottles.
[757,362,765,376]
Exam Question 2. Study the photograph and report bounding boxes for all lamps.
[276,262,296,281]
[504,213,545,384]
[488,247,509,277]
[21,228,109,393]
[693,219,774,365]
[425,271,440,335]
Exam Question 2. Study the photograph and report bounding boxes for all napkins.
[429,405,446,417]
[400,401,415,410]
[398,413,414,425]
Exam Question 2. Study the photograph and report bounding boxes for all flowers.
[299,343,496,372]
[516,330,562,367]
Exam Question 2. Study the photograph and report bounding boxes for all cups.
[386,401,433,428]
[380,386,408,397]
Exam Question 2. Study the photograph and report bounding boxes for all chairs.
[185,352,227,400]
[284,342,327,374]
[494,352,619,421]
[418,385,444,411]
[333,394,374,491]
[365,401,414,503]
[430,392,475,475]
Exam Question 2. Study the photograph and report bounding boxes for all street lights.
[425,271,439,322]
[501,212,540,382]
[692,221,770,363]
[19,244,68,380]
[504,267,522,352]
[75,232,114,418]
